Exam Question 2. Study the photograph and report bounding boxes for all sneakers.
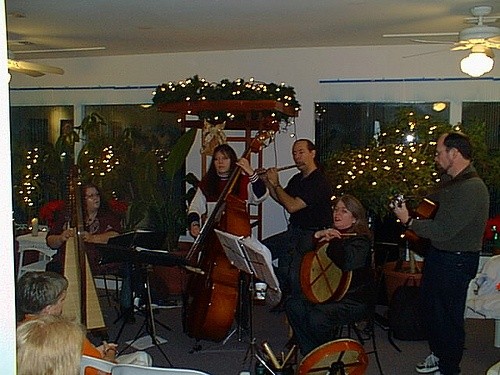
[416,352,439,373]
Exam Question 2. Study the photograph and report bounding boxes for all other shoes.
[120,306,135,324]
[278,293,290,312]
[283,344,300,351]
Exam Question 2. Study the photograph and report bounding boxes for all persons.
[257,138,332,314]
[285,194,377,358]
[45,182,138,326]
[393,131,490,375]
[15,268,119,375]
[187,144,270,340]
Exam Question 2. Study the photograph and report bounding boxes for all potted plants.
[325,104,500,314]
[121,128,199,296]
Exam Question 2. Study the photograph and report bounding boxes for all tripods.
[100,230,190,368]
[214,229,281,375]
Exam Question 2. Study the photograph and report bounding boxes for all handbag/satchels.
[389,276,429,342]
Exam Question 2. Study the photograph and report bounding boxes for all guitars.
[389,193,438,242]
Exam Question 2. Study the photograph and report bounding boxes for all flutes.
[256,163,305,176]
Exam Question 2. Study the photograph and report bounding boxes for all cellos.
[181,120,280,344]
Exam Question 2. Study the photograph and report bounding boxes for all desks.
[16,232,58,282]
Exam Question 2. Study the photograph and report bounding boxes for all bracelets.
[102,347,120,357]
[403,216,413,228]
[273,184,282,190]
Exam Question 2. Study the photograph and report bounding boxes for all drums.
[300,240,353,303]
[298,338,369,375]
